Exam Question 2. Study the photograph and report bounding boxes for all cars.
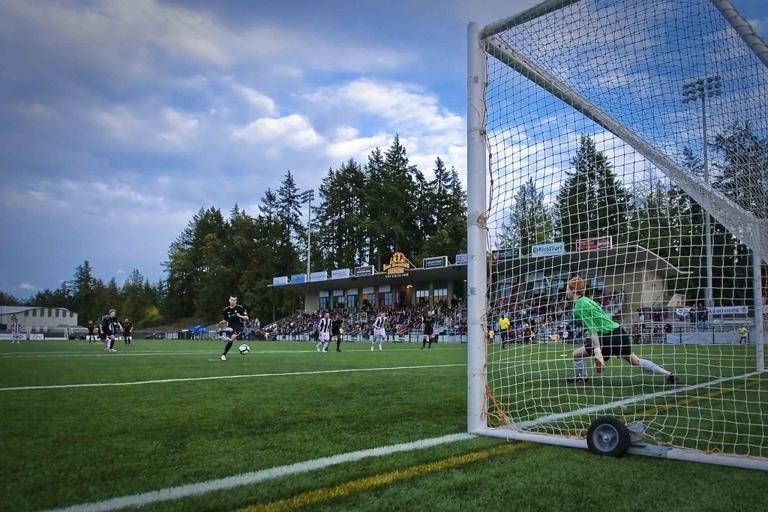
[145,331,166,340]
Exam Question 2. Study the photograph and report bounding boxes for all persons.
[564,277,678,385]
[689,303,708,321]
[10,318,22,344]
[237,293,468,353]
[217,294,249,360]
[87,309,134,352]
[486,289,670,359]
[739,325,748,343]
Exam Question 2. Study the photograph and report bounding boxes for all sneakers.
[221,355,226,360]
[667,373,679,384]
[219,335,231,342]
[316,345,342,352]
[568,373,589,383]
[371,345,382,351]
[105,346,117,352]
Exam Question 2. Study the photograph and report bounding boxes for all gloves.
[594,347,605,372]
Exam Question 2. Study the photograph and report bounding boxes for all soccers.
[239,344,250,354]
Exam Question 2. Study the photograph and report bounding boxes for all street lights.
[680,76,724,320]
[300,188,317,273]
